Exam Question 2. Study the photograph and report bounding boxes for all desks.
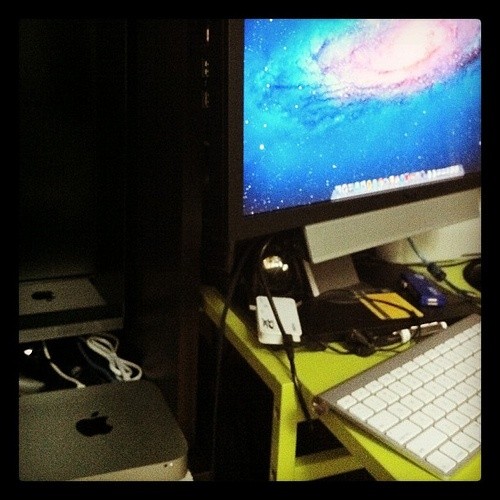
[176,258,481,482]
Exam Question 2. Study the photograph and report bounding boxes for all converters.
[19,274,124,343]
[19,380,188,482]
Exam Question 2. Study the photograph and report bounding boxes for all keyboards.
[313,314,481,481]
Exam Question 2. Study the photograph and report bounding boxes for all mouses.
[462,257,483,295]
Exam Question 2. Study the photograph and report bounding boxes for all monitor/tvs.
[207,18,481,244]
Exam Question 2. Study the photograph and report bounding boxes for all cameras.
[399,272,448,306]
